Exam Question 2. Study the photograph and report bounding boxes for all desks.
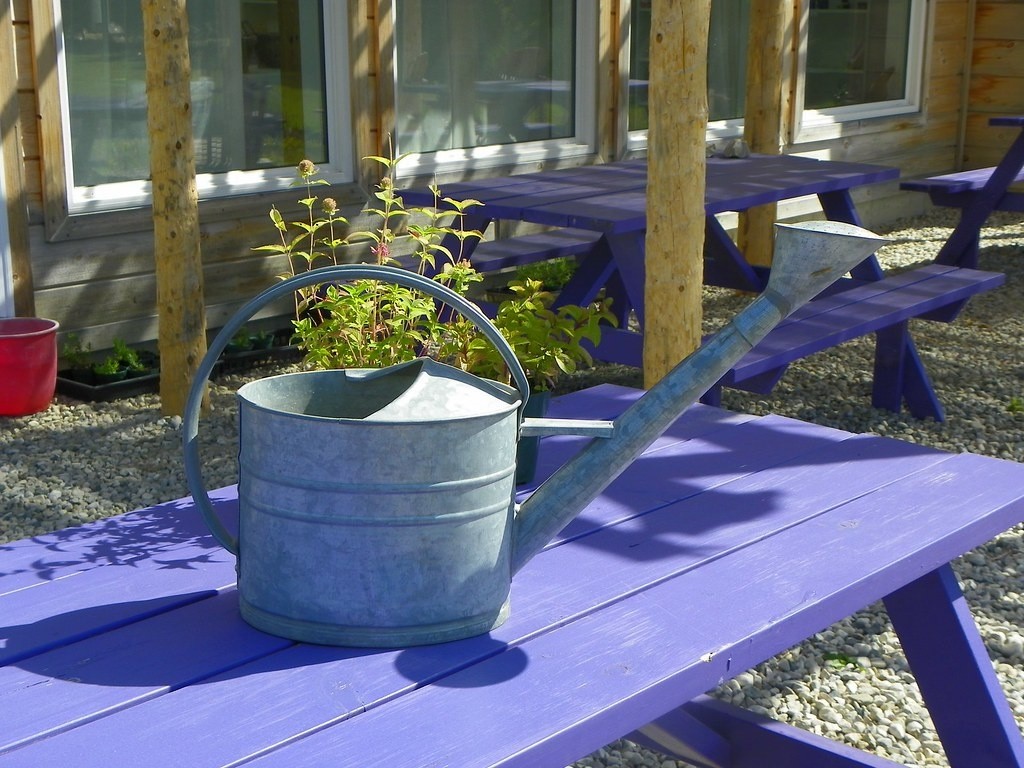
[0,381,1024,768]
[808,64,895,101]
[72,99,149,142]
[932,115,1024,264]
[397,75,648,152]
[377,144,948,425]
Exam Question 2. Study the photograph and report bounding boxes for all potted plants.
[252,143,620,489]
[55,317,276,403]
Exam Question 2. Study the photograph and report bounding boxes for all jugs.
[179,221,886,648]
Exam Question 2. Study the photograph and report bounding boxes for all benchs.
[393,122,565,149]
[387,162,1024,423]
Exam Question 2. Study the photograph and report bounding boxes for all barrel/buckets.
[0,317,59,416]
